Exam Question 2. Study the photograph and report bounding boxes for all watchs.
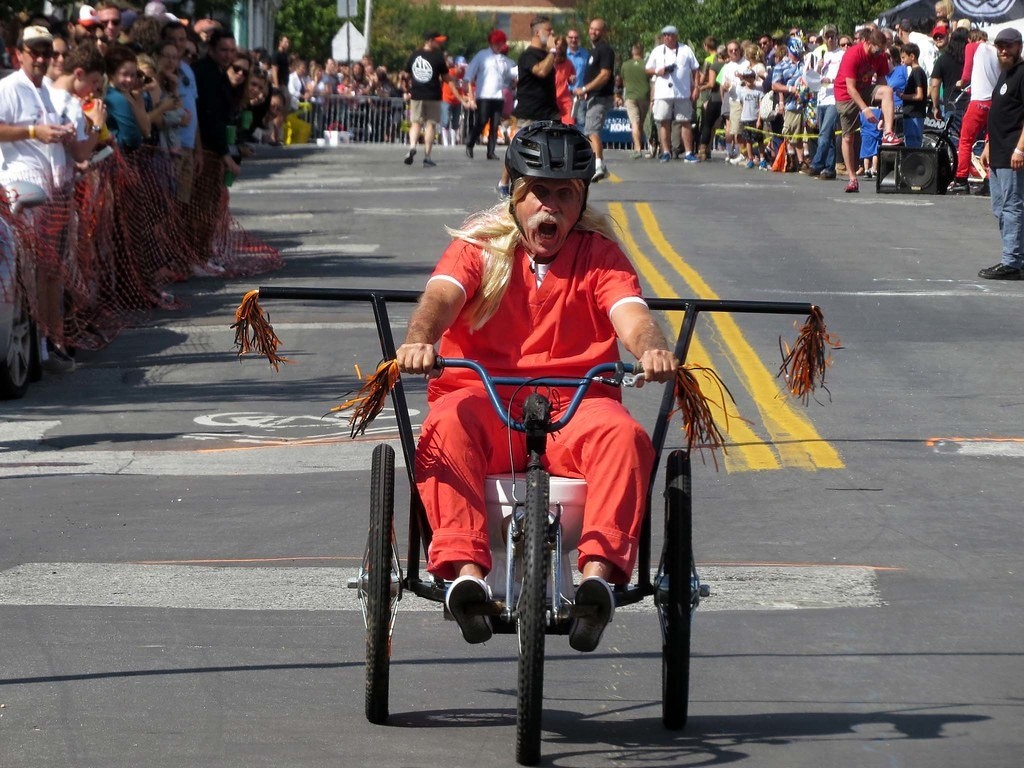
[90,124,102,134]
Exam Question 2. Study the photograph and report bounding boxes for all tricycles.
[256,286,814,765]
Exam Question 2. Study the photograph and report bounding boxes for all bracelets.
[1014,148,1024,156]
[789,86,792,94]
[862,106,867,113]
[900,92,903,99]
[830,78,834,84]
[549,47,558,56]
[655,69,659,76]
[582,87,588,93]
[28,125,34,139]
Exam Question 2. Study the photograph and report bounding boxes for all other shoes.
[881,131,904,146]
[403,148,417,164]
[591,165,610,183]
[632,147,990,197]
[423,157,436,166]
[445,574,494,645]
[568,575,615,653]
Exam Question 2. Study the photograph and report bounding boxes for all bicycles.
[921,85,990,195]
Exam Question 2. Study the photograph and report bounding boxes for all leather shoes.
[977,263,1023,280]
[466,146,474,158]
[487,154,500,161]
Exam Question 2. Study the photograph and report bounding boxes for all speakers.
[875,145,950,194]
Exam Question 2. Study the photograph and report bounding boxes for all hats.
[422,30,447,43]
[488,31,506,44]
[78,4,102,28]
[930,19,1023,49]
[141,2,168,18]
[15,24,53,48]
[661,25,678,36]
[822,23,837,36]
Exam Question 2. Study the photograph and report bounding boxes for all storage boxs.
[317,138,326,147]
[324,131,351,146]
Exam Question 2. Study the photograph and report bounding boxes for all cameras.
[665,65,674,73]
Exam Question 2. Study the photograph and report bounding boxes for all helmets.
[786,38,805,58]
[504,121,596,183]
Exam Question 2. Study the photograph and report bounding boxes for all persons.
[0,0,1024,373]
[395,121,680,652]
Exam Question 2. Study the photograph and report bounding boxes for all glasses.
[729,42,769,79]
[135,68,152,85]
[229,63,249,77]
[101,19,121,27]
